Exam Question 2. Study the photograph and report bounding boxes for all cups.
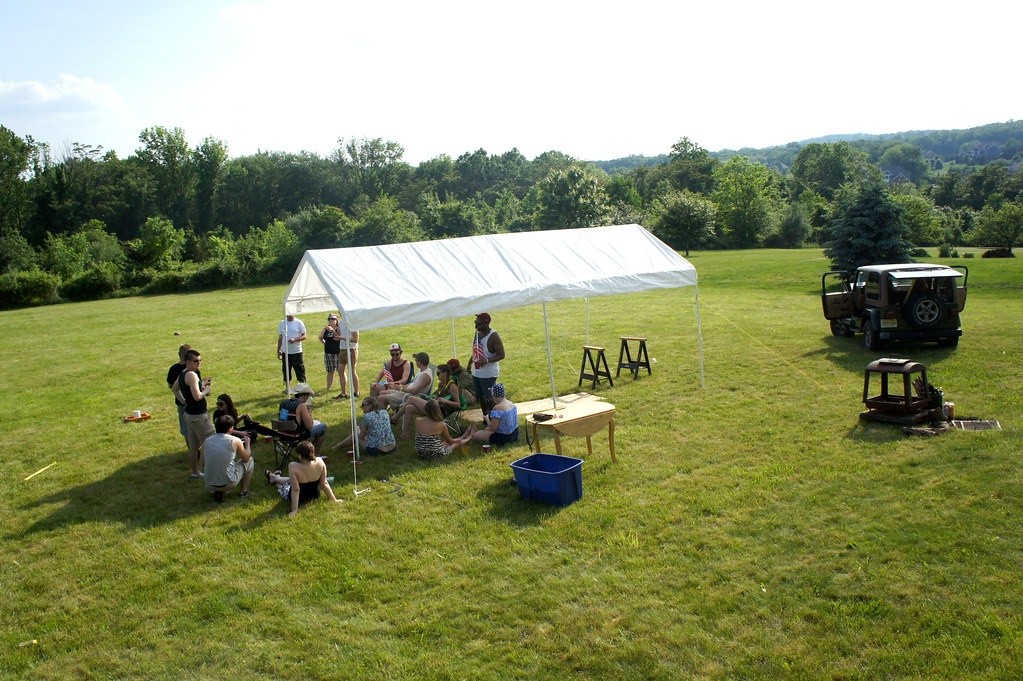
[462,446,469,455]
[474,360,482,369]
[348,451,353,457]
[132,410,141,418]
[265,436,272,443]
[483,444,490,453]
[327,476,335,488]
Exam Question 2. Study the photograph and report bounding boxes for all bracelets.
[400,386,405,391]
[486,358,489,363]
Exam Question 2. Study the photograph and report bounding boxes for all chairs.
[271,419,312,471]
[387,361,467,436]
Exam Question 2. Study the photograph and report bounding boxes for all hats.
[389,343,401,351]
[448,359,459,368]
[295,383,315,395]
[475,313,491,326]
[413,352,429,365]
[491,383,506,397]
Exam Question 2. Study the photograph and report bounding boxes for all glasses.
[328,318,336,321]
[362,404,368,407]
[437,371,442,376]
[216,402,224,407]
[193,360,201,363]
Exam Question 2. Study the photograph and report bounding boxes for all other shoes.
[191,471,204,478]
[354,393,360,397]
[239,490,254,498]
[332,394,347,399]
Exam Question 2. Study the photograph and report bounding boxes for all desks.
[523,400,620,464]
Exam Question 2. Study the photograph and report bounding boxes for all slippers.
[274,469,283,477]
[265,469,273,486]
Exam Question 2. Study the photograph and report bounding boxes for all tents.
[285,223,704,493]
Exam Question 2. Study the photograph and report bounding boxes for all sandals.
[396,435,411,441]
[390,417,397,425]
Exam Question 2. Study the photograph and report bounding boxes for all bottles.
[205,378,211,397]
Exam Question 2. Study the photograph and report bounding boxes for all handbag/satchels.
[534,413,553,421]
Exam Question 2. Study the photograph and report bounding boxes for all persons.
[390,364,461,424]
[279,382,327,460]
[370,343,412,397]
[318,313,340,391]
[198,415,254,503]
[447,359,476,405]
[327,316,360,398]
[466,313,505,423]
[264,440,344,517]
[414,399,476,458]
[213,394,260,443]
[277,315,306,394]
[377,352,433,410]
[171,350,215,478]
[473,384,518,445]
[332,396,396,455]
[167,344,202,456]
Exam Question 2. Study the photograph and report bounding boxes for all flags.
[473,330,484,369]
[384,362,395,382]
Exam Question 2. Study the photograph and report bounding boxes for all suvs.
[821,263,969,353]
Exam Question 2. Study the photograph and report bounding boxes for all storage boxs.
[509,452,583,510]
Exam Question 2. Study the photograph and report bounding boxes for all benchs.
[616,337,652,379]
[578,345,614,389]
